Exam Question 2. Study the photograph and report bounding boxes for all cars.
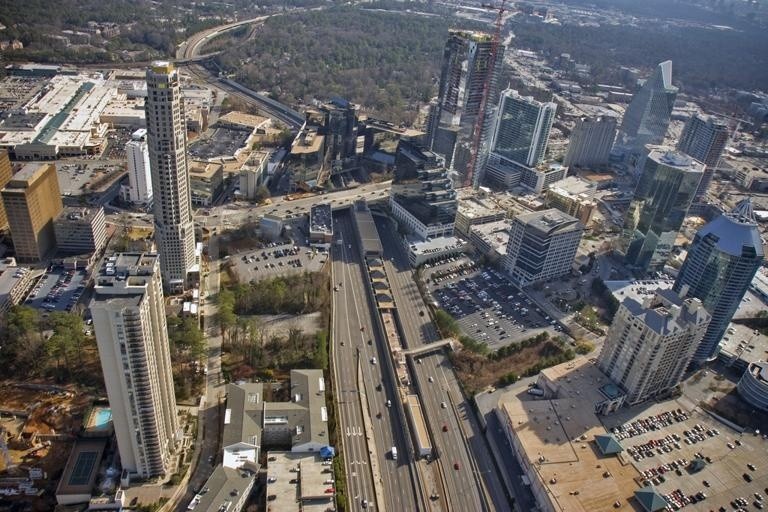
[13,263,97,320]
[325,275,461,512]
[234,240,305,275]
[425,250,564,345]
[609,405,768,511]
[627,273,768,362]
[199,452,304,504]
[497,351,606,508]
